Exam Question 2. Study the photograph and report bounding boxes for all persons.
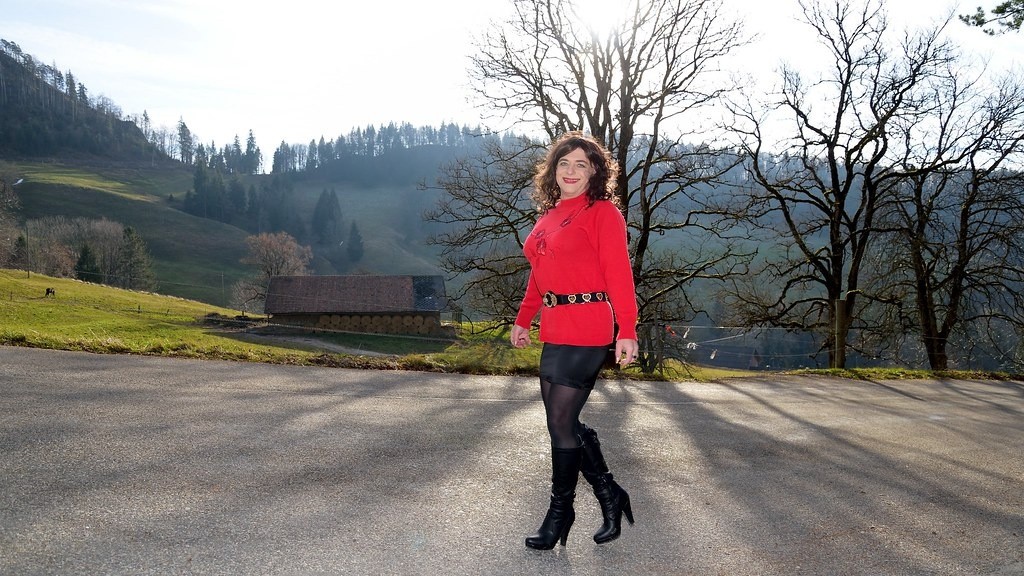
[509,131,639,552]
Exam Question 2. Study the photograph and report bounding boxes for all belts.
[541,290,608,308]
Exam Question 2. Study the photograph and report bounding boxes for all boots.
[526,423,635,551]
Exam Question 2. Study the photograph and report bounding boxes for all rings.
[518,338,525,341]
[631,354,638,360]
